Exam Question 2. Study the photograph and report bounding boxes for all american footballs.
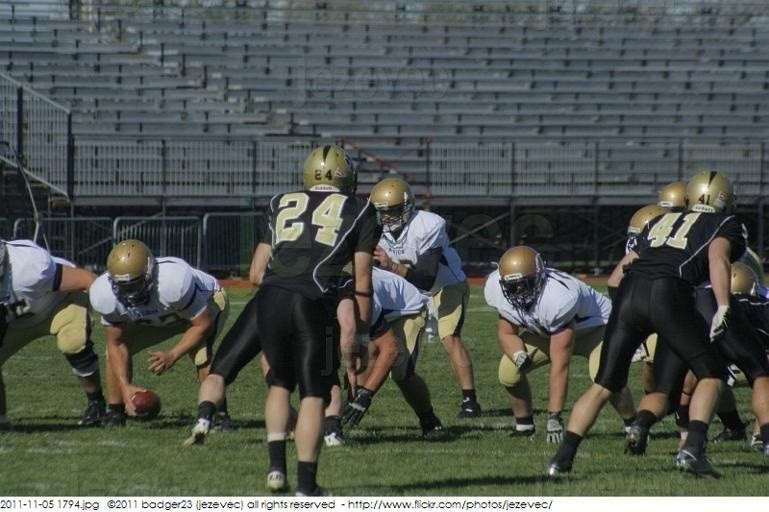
[130,392,161,418]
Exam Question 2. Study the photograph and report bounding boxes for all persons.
[89,239,233,432]
[0,239,106,432]
[186,144,768,496]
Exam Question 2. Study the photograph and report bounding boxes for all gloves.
[349,317,372,377]
[708,304,733,344]
[513,349,535,374]
[545,409,567,448]
[337,386,376,429]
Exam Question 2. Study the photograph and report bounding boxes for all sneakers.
[674,448,728,481]
[504,424,538,443]
[208,411,242,434]
[293,485,335,497]
[706,426,748,443]
[322,426,347,448]
[101,409,131,430]
[620,423,651,457]
[752,431,769,459]
[545,450,574,480]
[74,398,106,430]
[457,396,480,419]
[180,417,210,448]
[420,417,446,441]
[265,465,291,494]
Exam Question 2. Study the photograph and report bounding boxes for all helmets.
[369,175,417,234]
[301,143,359,196]
[105,238,159,311]
[497,245,547,313]
[730,261,757,298]
[682,167,735,216]
[657,179,688,212]
[625,203,672,256]
